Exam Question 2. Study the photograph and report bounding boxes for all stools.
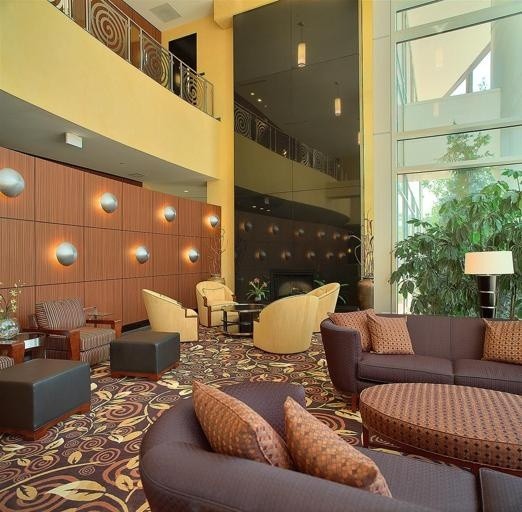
[108,330,181,379]
[0,353,94,441]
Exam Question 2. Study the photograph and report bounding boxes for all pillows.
[365,310,417,357]
[282,393,394,500]
[190,379,292,471]
[328,308,377,352]
[476,319,521,366]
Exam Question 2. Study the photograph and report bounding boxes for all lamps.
[333,81,343,119]
[460,251,515,318]
[295,21,309,69]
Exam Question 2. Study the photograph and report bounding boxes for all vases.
[254,293,262,301]
[207,273,225,286]
[0,318,21,340]
[357,276,375,312]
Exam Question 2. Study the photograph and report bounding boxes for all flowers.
[245,277,272,302]
[1,278,25,319]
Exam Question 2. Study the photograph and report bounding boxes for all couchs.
[137,380,522,512]
[314,305,522,395]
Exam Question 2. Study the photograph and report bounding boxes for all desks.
[0,331,50,367]
[86,306,112,328]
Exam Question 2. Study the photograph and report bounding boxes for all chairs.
[278,280,311,296]
[140,287,200,343]
[195,281,237,329]
[0,339,26,370]
[252,295,322,356]
[308,280,342,334]
[31,297,123,367]
